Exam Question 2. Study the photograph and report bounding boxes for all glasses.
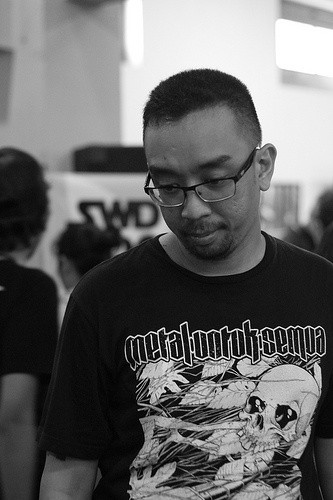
[144,147,260,207]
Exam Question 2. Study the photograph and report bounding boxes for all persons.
[39,68,333,500]
[54,223,111,326]
[0,149,61,500]
